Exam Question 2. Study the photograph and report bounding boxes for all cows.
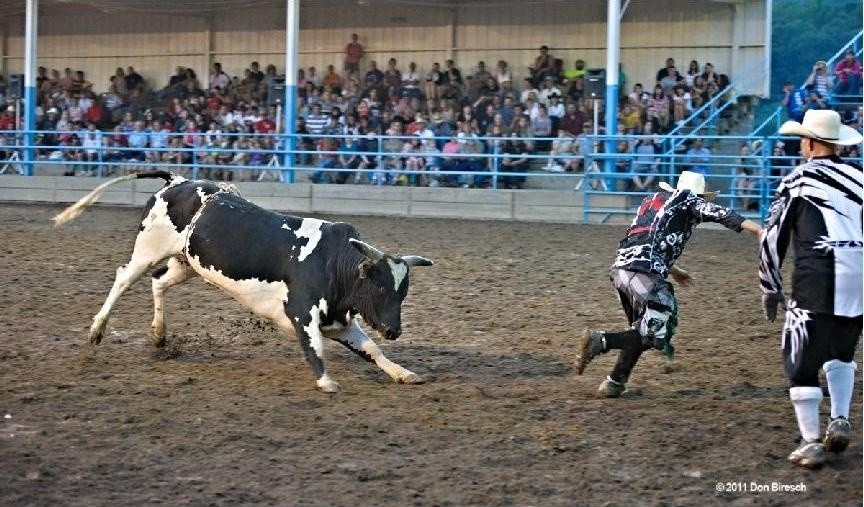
[49,170,434,395]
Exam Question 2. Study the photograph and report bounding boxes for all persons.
[616,50,863,212]
[2,62,287,179]
[754,108,862,467]
[573,169,764,399]
[296,30,608,189]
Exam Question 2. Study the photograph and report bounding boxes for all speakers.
[7,73,24,99]
[583,68,607,99]
[268,84,286,105]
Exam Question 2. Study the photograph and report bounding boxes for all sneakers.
[788,414,850,467]
[572,326,628,398]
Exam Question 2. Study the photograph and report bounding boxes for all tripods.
[573,99,609,191]
[257,105,285,183]
[0,99,25,176]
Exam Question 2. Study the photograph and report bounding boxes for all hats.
[777,108,863,146]
[659,170,719,202]
